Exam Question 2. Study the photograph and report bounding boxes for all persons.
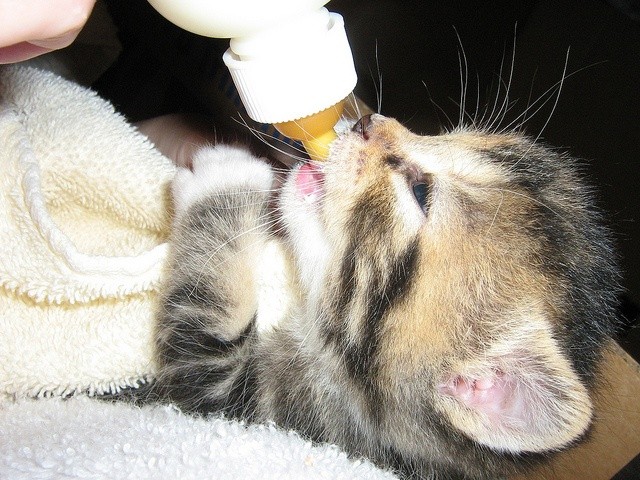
[0,1,220,176]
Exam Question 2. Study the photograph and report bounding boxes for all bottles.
[149,0,360,161]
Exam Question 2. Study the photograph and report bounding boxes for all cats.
[86,18,640,480]
